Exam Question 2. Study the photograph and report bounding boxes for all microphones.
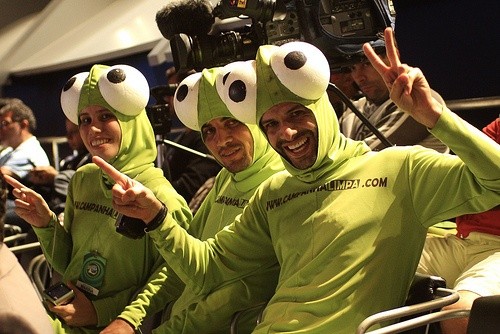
[155,0,215,40]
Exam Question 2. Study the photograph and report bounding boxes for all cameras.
[42,281,75,307]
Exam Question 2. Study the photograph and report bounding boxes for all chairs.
[354,275,500,334]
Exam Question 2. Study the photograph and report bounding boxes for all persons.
[92,27,500,334]
[0,59,500,334]
